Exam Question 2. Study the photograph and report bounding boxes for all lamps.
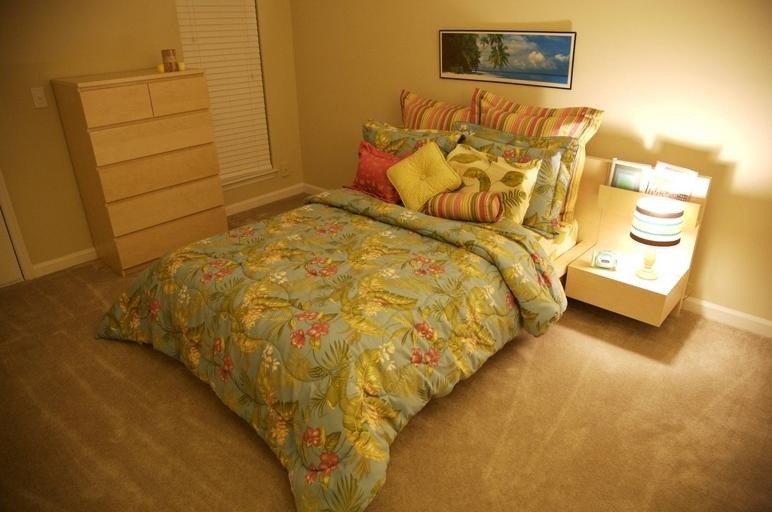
[625,197,686,280]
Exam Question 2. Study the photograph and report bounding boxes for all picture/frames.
[437,29,577,93]
[608,155,651,194]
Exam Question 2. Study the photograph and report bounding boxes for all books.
[607,159,698,201]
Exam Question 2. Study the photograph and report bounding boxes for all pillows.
[386,140,463,214]
[347,139,410,205]
[469,88,605,240]
[364,118,463,160]
[401,90,472,136]
[451,122,580,236]
[445,143,543,224]
[426,190,503,223]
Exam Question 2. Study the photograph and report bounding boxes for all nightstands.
[561,182,709,335]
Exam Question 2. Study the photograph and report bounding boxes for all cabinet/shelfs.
[49,65,231,277]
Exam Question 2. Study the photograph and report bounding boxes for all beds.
[91,151,610,510]
